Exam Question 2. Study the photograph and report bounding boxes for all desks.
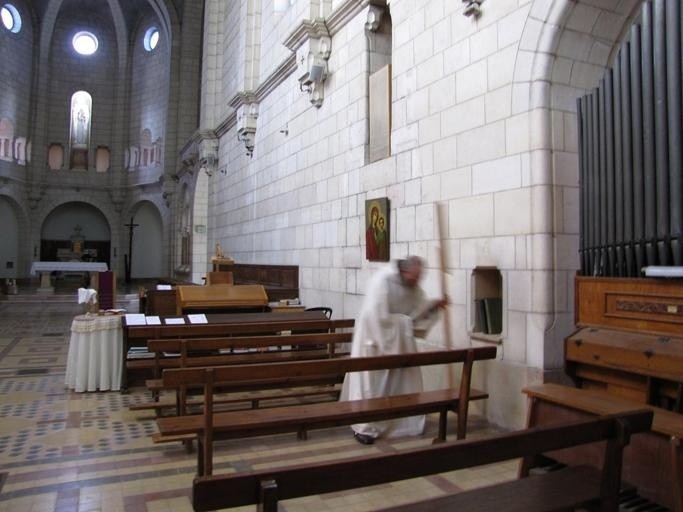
[65,312,123,392]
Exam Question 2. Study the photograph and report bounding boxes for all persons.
[338,256,450,445]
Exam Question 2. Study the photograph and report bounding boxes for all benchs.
[121,307,655,512]
[520,382,682,512]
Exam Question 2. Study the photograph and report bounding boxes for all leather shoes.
[355,434,373,444]
